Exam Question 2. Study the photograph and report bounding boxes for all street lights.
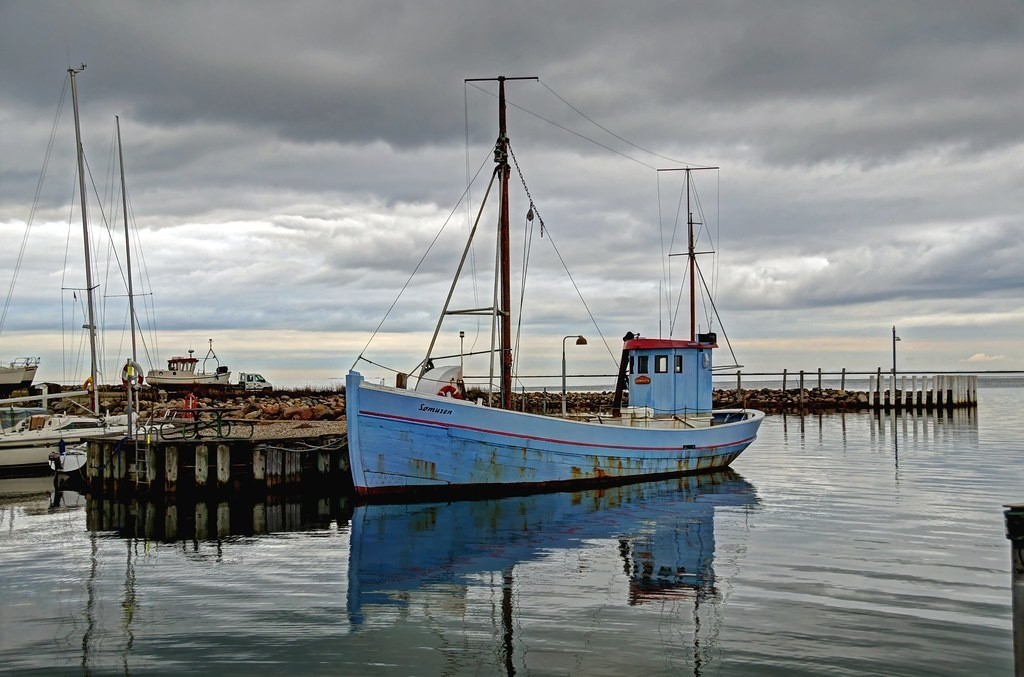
[561,335,587,417]
[891,324,901,392]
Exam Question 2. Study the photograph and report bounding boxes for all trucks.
[229,371,272,392]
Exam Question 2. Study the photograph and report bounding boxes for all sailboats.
[0,61,175,506]
[344,75,768,503]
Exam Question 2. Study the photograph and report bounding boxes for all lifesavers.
[122,362,144,391]
[184,394,196,418]
[82,377,94,398]
[438,385,461,399]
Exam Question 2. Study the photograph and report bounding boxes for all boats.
[145,338,232,386]
[346,463,764,676]
[3,357,40,390]
[0,356,28,393]
